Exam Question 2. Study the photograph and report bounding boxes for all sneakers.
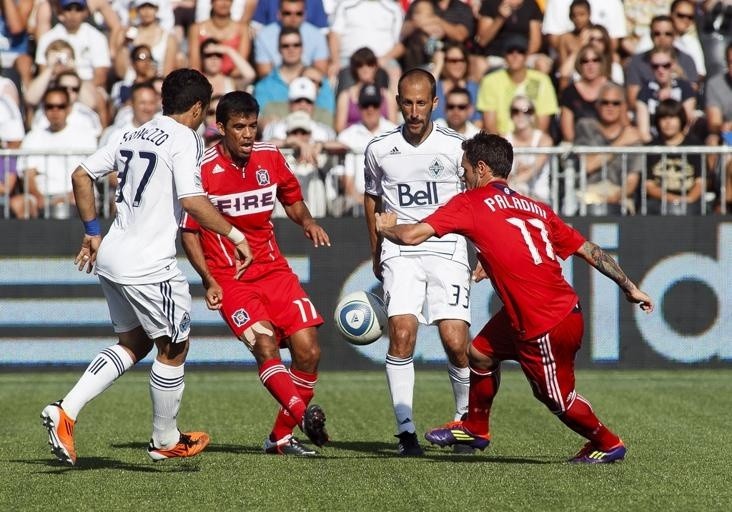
[146,430,210,463]
[39,400,77,466]
[452,443,479,453]
[394,430,424,455]
[565,435,627,464]
[301,404,330,447]
[425,421,490,451]
[262,432,320,456]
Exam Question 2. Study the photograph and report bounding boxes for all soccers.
[334,291,387,345]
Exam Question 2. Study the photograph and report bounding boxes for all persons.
[0,0,731,218]
[373,131,655,466]
[179,88,333,458]
[362,66,489,458]
[38,66,255,468]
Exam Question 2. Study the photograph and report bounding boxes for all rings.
[83,253,91,260]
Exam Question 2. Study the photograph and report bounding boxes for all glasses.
[45,104,66,110]
[281,11,304,16]
[445,12,694,116]
[281,43,300,49]
[62,87,79,92]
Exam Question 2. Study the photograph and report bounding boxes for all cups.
[705,192,715,216]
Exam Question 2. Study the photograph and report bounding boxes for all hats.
[58,0,87,9]
[288,77,317,104]
[284,110,313,136]
[358,83,382,107]
[132,1,160,10]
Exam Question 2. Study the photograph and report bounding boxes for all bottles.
[669,199,682,217]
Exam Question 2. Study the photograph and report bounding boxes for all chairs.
[1,0,731,216]
